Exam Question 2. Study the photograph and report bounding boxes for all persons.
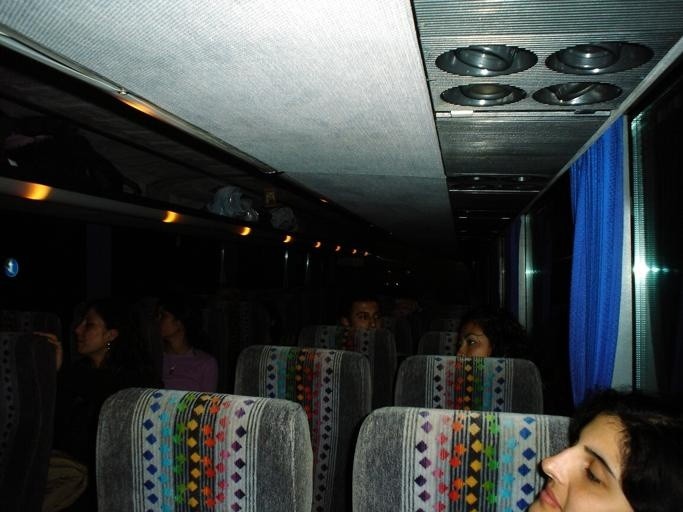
[526,387,683,512]
[148,290,219,392]
[30,297,154,512]
[337,287,526,361]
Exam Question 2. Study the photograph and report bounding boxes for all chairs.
[352,407,569,512]
[392,355,545,418]
[232,345,373,510]
[265,319,462,405]
[0,306,70,509]
[98,388,312,512]
